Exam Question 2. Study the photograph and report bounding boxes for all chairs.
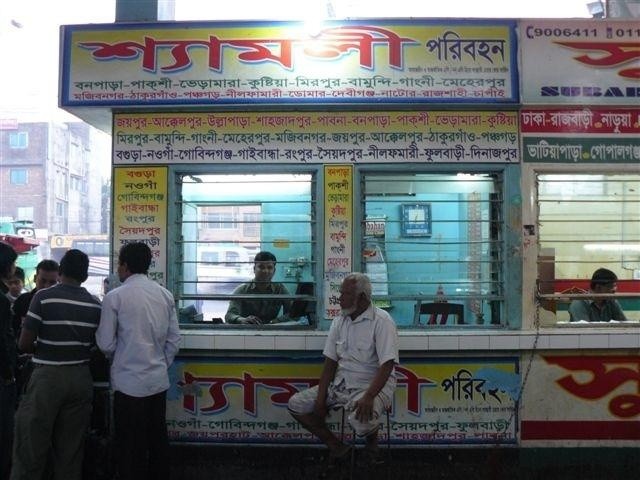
[414,303,464,325]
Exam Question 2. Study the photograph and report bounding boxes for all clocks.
[401,202,432,237]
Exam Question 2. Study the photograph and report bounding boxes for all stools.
[342,408,390,480]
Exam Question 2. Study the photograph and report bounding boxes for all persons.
[286,270,400,472]
[1,242,111,479]
[95,242,183,480]
[225,251,301,326]
[566,266,628,323]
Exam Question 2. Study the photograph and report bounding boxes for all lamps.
[587,0,605,19]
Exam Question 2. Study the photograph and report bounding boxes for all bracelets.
[269,320,273,323]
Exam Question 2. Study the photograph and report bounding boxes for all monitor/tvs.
[289,283,315,325]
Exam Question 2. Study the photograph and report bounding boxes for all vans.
[197,246,255,291]
